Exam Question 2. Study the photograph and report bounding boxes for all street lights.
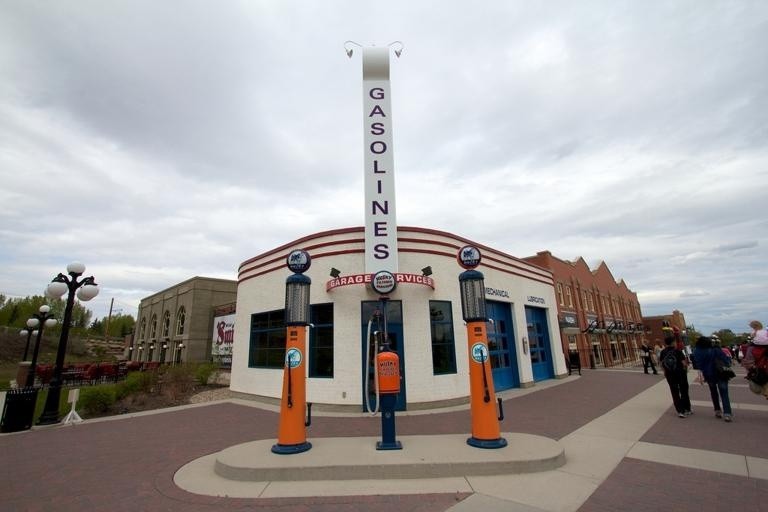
[19,326,39,362]
[37,261,100,426]
[24,304,58,390]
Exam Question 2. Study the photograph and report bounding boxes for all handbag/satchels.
[712,355,737,380]
[640,349,649,358]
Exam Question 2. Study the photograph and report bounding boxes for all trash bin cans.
[2,388,40,431]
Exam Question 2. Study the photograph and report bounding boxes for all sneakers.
[676,409,733,421]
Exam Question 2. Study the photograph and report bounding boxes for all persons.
[639,321,768,422]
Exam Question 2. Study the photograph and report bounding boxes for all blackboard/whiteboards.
[567,352,581,376]
